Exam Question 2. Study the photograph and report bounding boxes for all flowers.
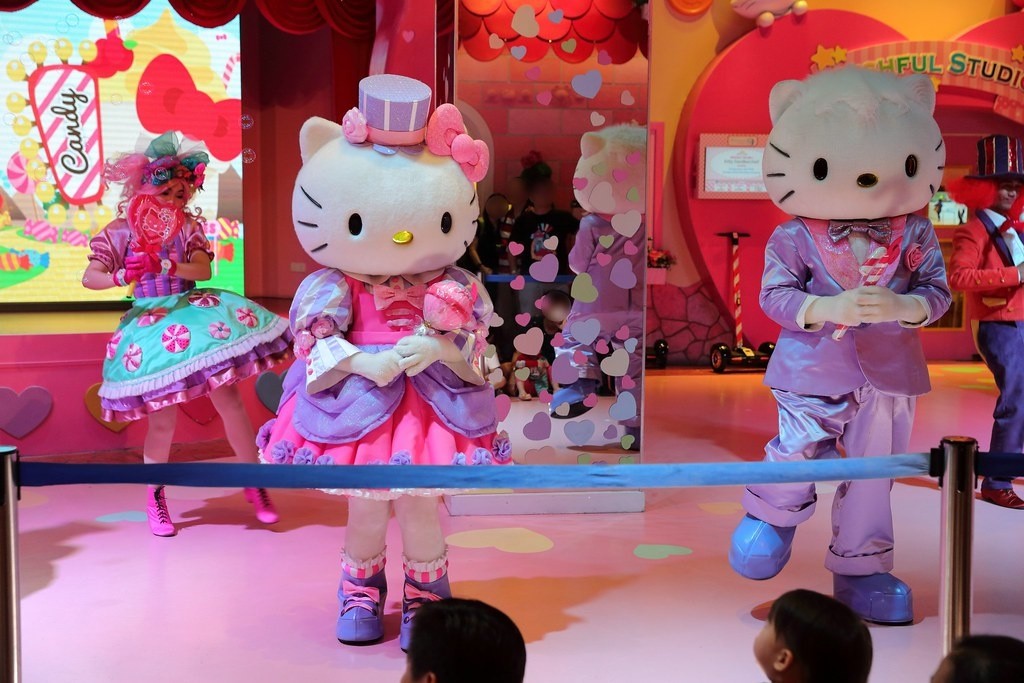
[521,151,542,168]
[646,237,678,271]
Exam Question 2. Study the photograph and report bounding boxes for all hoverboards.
[711,230,780,374]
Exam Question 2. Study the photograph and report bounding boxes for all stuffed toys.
[255,73,516,654]
[550,121,645,453]
[726,63,954,630]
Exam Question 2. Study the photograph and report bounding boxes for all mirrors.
[434,0,655,514]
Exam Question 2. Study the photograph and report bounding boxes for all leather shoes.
[981,487,1024,509]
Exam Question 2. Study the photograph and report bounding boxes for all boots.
[244,487,279,524]
[145,484,175,537]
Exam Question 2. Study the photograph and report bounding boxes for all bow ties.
[998,213,1024,233]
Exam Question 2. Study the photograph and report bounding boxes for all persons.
[400,597,526,683]
[931,634,1024,683]
[751,588,874,683]
[467,156,591,402]
[945,133,1024,509]
[81,154,284,537]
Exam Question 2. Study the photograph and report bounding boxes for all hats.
[963,133,1024,180]
[101,131,210,203]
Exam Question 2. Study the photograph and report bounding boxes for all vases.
[647,267,667,285]
